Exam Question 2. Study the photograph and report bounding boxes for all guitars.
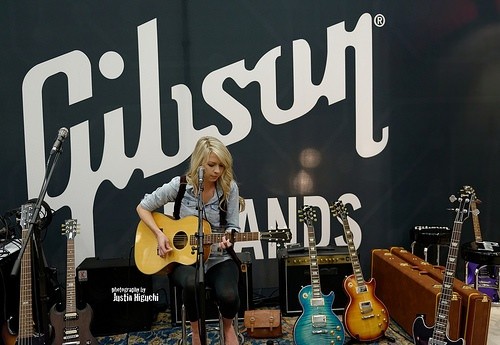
[134,211,293,277]
[0,204,53,345]
[329,201,390,342]
[48,219,99,345]
[463,196,500,302]
[411,186,480,345]
[292,205,346,345]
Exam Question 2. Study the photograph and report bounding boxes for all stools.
[182,287,239,345]
[411,224,451,265]
[461,240,500,300]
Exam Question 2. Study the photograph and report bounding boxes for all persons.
[136,136,240,345]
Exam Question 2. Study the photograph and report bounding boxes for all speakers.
[277,246,361,317]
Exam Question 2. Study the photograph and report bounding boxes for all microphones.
[198,166,205,182]
[49,126,68,155]
